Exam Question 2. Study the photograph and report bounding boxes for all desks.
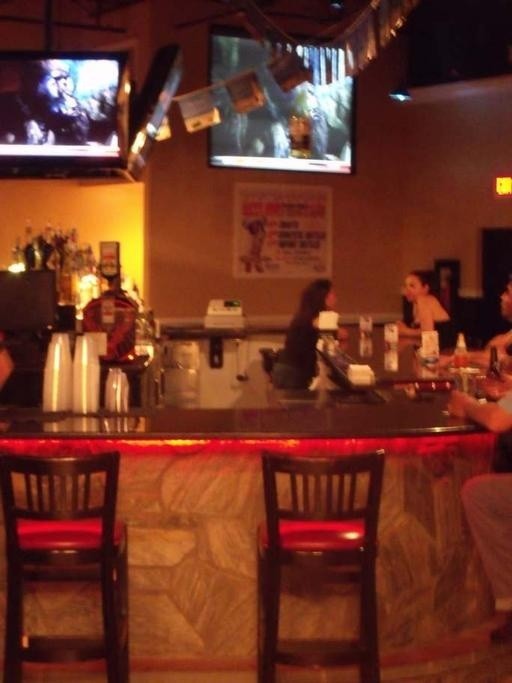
[315,325,460,401]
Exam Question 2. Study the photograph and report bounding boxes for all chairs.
[248,443,387,682]
[258,345,319,419]
[0,448,133,682]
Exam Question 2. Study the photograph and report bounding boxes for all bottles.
[453,332,470,368]
[484,345,506,401]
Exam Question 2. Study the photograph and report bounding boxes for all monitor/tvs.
[0,49,132,180]
[207,21,357,177]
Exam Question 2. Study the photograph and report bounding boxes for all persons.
[1,60,119,146]
[445,363,511,649]
[270,274,341,395]
[426,276,511,374]
[392,269,462,353]
[81,262,147,409]
[0,267,56,409]
